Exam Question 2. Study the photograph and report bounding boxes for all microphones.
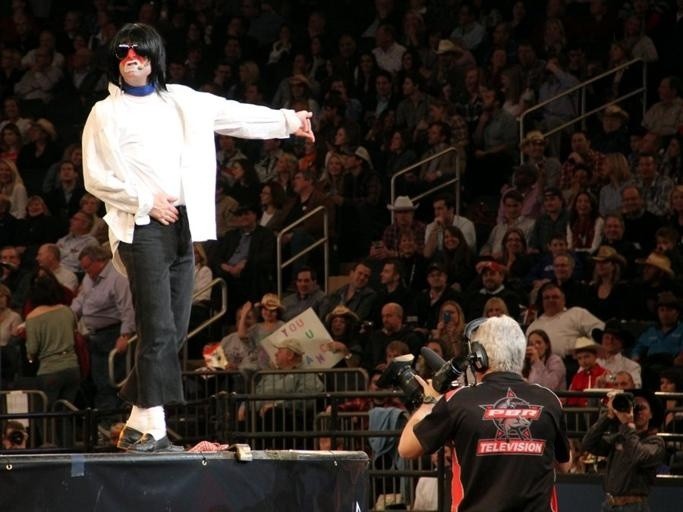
[137,59,150,71]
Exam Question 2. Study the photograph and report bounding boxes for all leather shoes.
[115,424,185,455]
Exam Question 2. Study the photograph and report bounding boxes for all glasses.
[113,43,149,60]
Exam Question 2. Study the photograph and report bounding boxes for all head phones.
[462,320,488,373]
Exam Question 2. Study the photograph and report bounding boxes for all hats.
[267,334,308,361]
[590,318,638,353]
[350,144,375,174]
[646,290,682,312]
[600,103,631,123]
[567,333,607,360]
[633,250,677,280]
[227,199,265,220]
[623,383,666,433]
[383,194,424,215]
[516,128,551,150]
[589,243,630,267]
[251,292,291,314]
[321,302,362,326]
[428,38,465,59]
[425,262,449,273]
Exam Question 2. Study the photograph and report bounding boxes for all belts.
[602,491,648,506]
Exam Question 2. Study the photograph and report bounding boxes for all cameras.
[362,320,374,327]
[605,374,617,382]
[443,312,450,321]
[611,392,634,411]
